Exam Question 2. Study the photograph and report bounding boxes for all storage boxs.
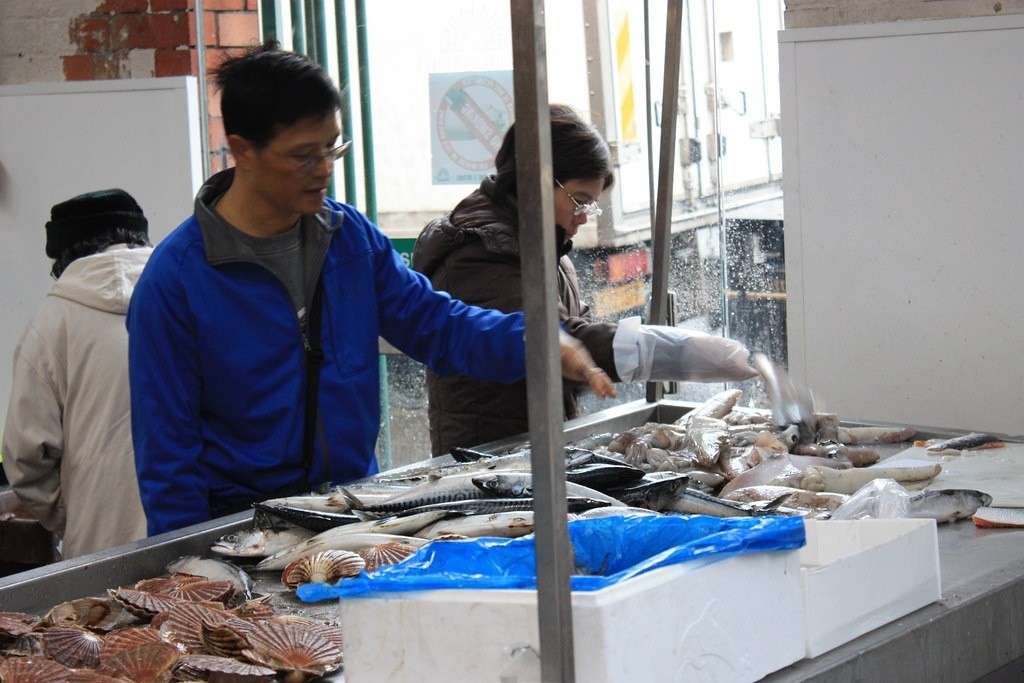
[338,513,809,683]
[807,517,941,661]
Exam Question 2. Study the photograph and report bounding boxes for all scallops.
[0,579,343,683]
[282,550,362,589]
[360,541,418,571]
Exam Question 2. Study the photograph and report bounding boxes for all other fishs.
[926,432,1005,452]
[169,439,793,590]
[908,487,993,524]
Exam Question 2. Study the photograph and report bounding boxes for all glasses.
[256,130,354,171]
[553,178,603,217]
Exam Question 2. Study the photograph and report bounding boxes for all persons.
[410,102,761,459]
[0,188,156,561]
[125,46,615,538]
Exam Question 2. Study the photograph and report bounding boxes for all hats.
[45,188,149,259]
